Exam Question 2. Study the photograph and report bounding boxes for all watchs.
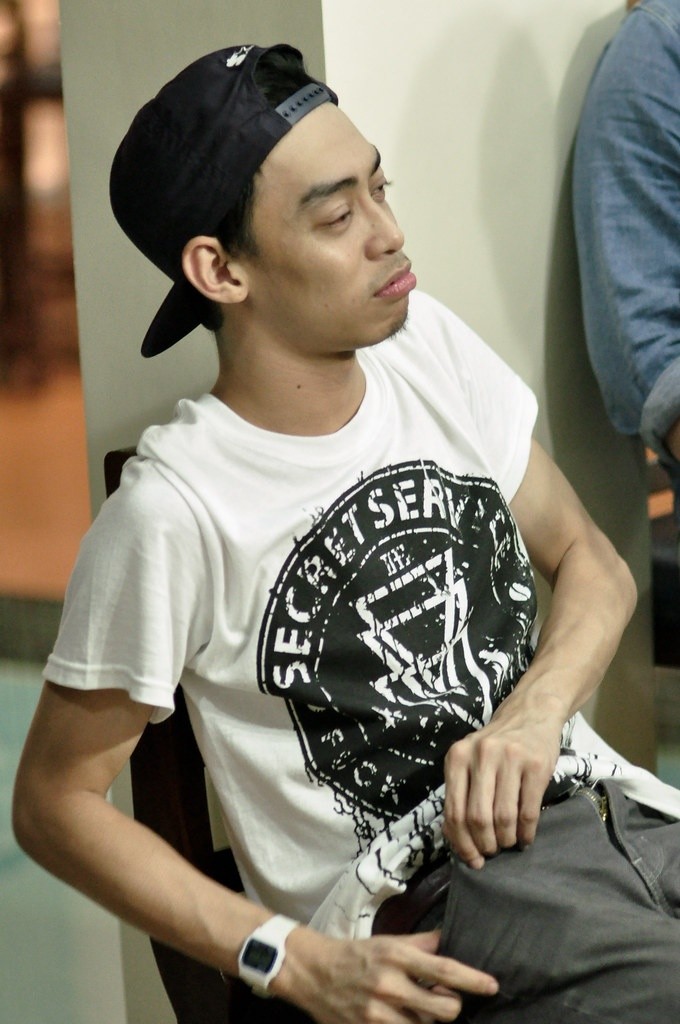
[237,913,299,1001]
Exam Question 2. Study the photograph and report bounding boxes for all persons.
[13,44,679,1024]
[571,0,680,500]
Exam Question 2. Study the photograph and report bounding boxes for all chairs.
[97,439,332,1024]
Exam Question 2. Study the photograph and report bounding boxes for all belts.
[373,858,451,936]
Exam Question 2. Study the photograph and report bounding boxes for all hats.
[109,41,338,357]
